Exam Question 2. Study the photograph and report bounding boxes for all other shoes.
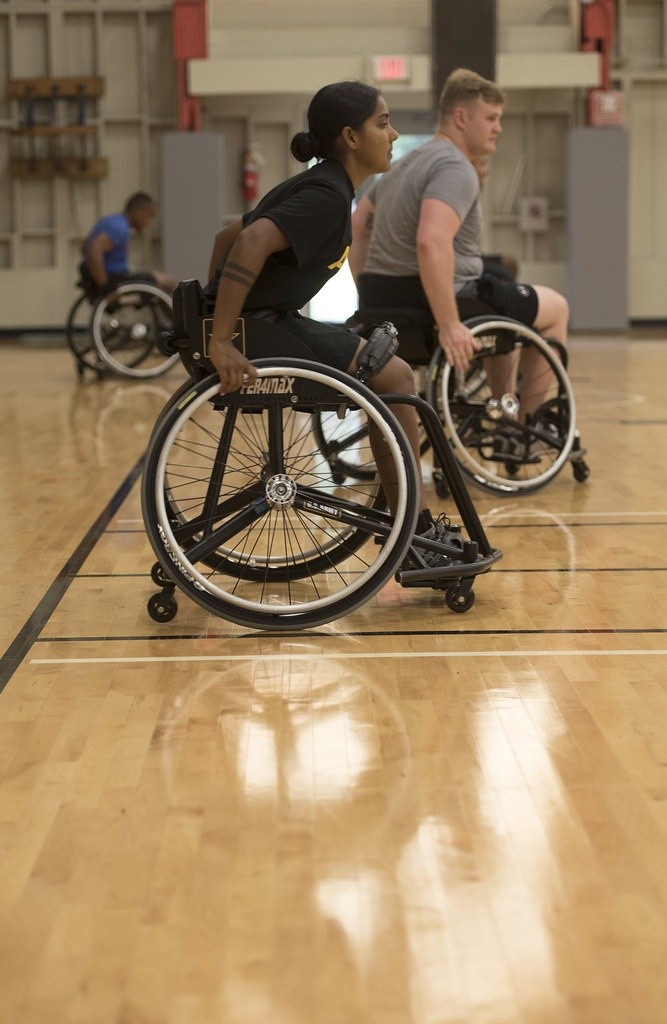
[402,512,466,572]
[492,434,556,458]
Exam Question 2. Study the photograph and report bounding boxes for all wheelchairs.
[138,276,504,634]
[64,261,188,379]
[312,273,593,498]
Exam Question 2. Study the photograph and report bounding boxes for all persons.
[207,78,483,567]
[77,193,178,332]
[344,68,580,457]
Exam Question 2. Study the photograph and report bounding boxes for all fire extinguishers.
[241,150,265,201]
